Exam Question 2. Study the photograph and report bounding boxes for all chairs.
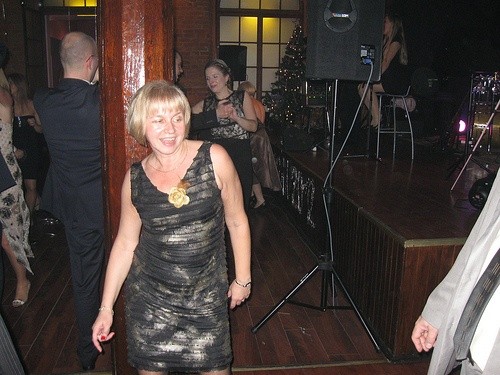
[366,73,414,162]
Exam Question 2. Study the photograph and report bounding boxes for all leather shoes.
[83,364,95,370]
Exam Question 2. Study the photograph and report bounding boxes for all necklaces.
[148,140,188,172]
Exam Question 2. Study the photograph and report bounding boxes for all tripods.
[251,79,391,363]
[444,73,493,180]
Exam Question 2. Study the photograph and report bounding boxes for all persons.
[173,52,282,234]
[0,31,42,375]
[92,79,252,375]
[32,32,106,373]
[410,166,500,375]
[358,12,411,131]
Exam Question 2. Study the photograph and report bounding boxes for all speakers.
[304,0,386,82]
[219,45,247,81]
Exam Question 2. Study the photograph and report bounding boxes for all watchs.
[235,278,252,287]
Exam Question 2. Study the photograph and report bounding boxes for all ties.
[453,247,500,359]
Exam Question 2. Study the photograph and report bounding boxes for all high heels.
[12,280,31,307]
[254,200,266,209]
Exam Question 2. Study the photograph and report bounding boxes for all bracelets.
[98,306,114,316]
